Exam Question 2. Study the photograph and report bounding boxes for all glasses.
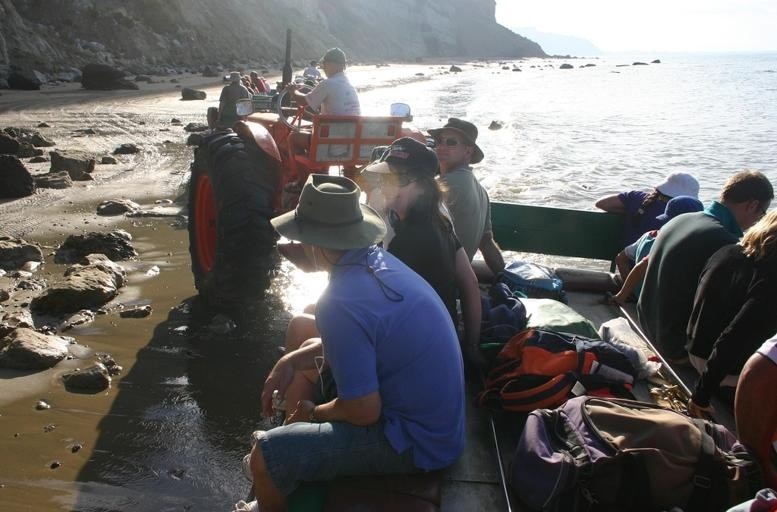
[434,135,461,146]
[389,176,416,187]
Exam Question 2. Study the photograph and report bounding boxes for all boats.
[275,196,771,512]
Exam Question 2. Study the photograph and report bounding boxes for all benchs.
[294,115,401,180]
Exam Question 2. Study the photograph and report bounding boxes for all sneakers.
[232,499,259,512]
[243,453,253,483]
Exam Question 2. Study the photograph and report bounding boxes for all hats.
[360,145,389,182]
[427,118,484,163]
[655,173,699,197]
[319,48,345,64]
[366,137,439,175]
[229,72,242,82]
[270,174,387,249]
[656,196,703,222]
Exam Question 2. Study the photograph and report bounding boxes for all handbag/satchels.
[472,260,766,512]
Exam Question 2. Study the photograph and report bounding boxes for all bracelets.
[467,343,480,348]
[308,409,322,423]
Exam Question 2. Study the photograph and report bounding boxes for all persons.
[595,173,698,268]
[428,116,503,275]
[282,48,360,189]
[220,62,323,120]
[610,196,702,306]
[285,137,488,371]
[233,174,465,511]
[735,334,777,488]
[685,207,777,418]
[637,170,775,365]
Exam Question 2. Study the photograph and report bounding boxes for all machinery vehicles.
[185,28,432,306]
